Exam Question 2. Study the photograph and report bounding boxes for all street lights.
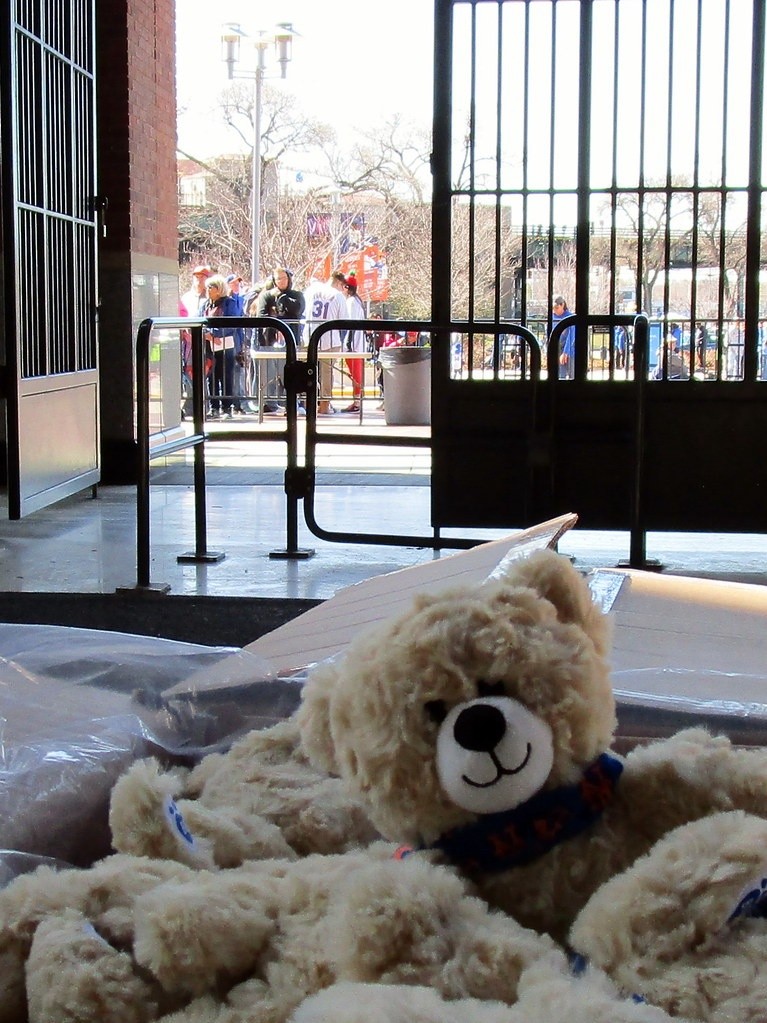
[221,22,298,285]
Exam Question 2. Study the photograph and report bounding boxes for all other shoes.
[316,408,339,416]
[204,403,306,422]
[340,403,360,414]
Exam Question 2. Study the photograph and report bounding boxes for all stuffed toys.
[0,548,767,1023]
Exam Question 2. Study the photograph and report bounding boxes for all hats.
[193,267,209,278]
[345,277,358,287]
[552,296,563,308]
[227,274,242,285]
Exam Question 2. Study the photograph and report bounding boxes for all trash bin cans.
[161,337,181,427]
[379,346,431,426]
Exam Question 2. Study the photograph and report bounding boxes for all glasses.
[208,286,216,289]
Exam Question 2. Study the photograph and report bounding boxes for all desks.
[251,351,373,426]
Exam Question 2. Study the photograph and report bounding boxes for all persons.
[672,323,681,355]
[724,317,767,380]
[452,334,464,378]
[514,336,523,371]
[696,322,708,366]
[552,296,575,380]
[365,314,430,410]
[180,267,365,420]
[615,326,626,369]
